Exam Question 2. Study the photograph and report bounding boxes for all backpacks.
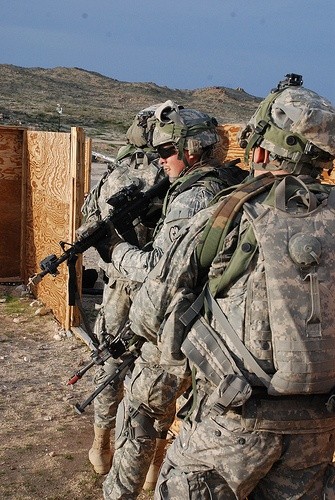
[230,187,335,402]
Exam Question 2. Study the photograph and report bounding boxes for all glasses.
[156,144,176,160]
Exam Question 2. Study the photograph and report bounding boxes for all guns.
[64,319,147,415]
[26,176,172,305]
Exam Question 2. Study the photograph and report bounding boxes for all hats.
[236,73,335,176]
[125,102,164,148]
[152,99,220,156]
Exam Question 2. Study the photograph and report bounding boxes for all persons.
[92,109,253,500]
[131,74,335,500]
[82,101,181,475]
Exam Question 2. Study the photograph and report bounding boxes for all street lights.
[56,104,63,132]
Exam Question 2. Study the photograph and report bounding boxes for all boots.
[89,427,112,475]
[141,437,170,491]
